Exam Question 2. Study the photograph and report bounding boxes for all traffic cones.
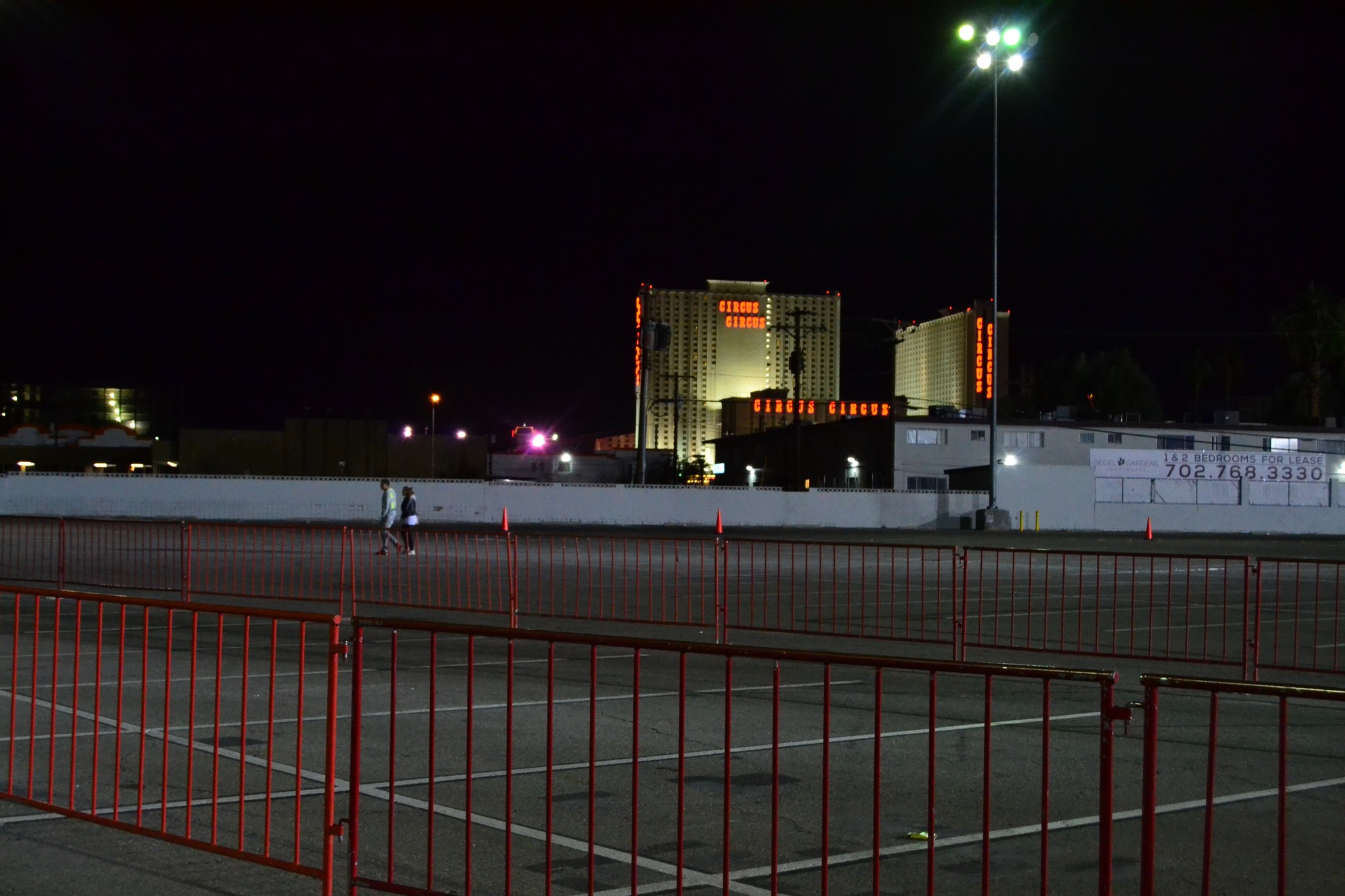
[713,510,723,534]
[499,505,510,532]
[1144,517,1155,540]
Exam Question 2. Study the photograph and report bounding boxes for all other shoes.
[402,547,410,554]
[397,545,402,554]
[408,550,415,555]
[375,550,388,555]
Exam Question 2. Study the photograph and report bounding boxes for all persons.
[400,486,418,556]
[375,480,403,555]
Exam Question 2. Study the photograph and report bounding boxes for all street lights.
[959,21,1028,532]
[431,393,440,478]
